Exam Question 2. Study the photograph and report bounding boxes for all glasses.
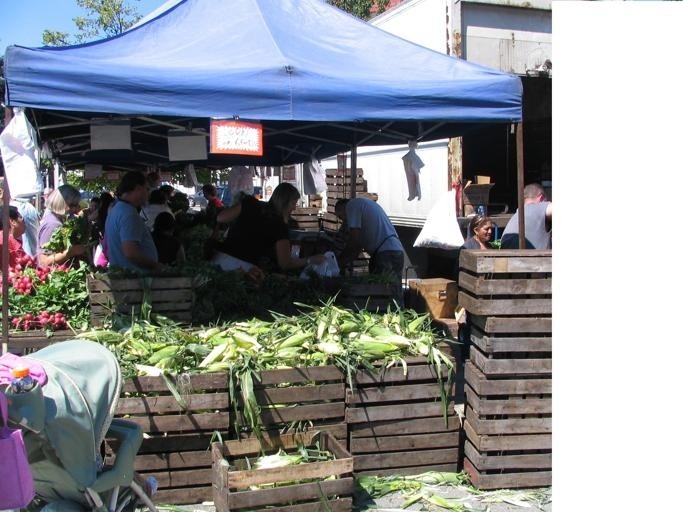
[68,203,81,209]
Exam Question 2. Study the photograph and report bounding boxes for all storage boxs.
[408,277,458,319]
[462,175,495,218]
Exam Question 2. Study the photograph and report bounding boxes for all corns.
[76,302,466,373]
[412,470,472,486]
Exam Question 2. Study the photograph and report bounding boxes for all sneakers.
[137,477,158,505]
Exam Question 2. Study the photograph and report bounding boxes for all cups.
[10,366,33,394]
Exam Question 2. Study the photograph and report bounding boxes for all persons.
[334,197,405,308]
[456,215,495,338]
[500,183,552,250]
